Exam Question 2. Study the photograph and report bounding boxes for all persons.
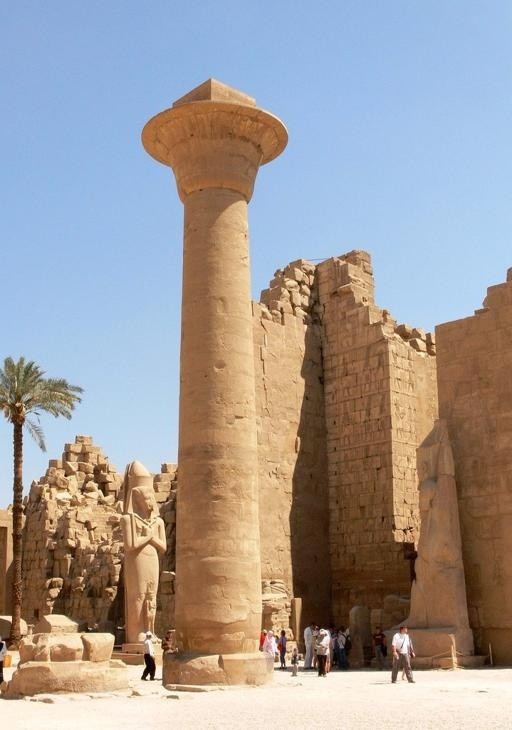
[391,626,416,683]
[161,632,171,651]
[141,632,156,680]
[0,635,8,685]
[372,626,386,668]
[262,622,351,677]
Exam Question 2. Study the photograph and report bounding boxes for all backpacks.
[342,634,352,655]
